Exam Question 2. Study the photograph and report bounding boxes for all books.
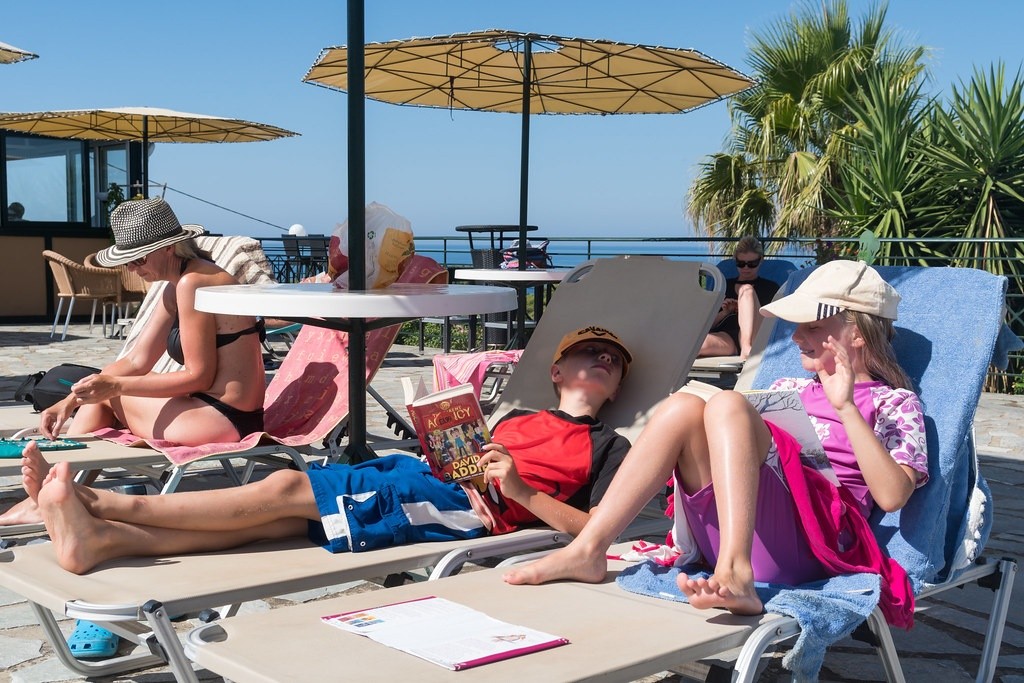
[400,375,494,485]
[669,379,842,489]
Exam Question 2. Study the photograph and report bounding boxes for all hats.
[758,260,902,324]
[96,198,204,267]
[553,324,632,384]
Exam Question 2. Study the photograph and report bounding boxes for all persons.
[0,196,928,622]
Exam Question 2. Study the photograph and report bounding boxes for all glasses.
[735,256,761,269]
[123,255,147,267]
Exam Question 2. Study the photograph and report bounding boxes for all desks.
[194,282,519,463]
[454,269,572,352]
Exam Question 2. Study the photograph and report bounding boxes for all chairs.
[686,259,799,389]
[41,250,151,341]
[282,234,328,284]
[0,235,274,441]
[0,256,726,683]
[180,263,1024,683]
[419,267,476,356]
[0,257,448,550]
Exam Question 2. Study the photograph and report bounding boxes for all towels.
[118,234,278,374]
[615,266,1024,683]
[86,255,446,467]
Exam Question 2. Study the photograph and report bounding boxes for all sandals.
[66,619,119,657]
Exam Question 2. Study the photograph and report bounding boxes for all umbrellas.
[301,29,762,351]
[0,41,302,200]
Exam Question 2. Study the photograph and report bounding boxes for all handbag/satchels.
[328,200,415,290]
[14,370,46,402]
[33,363,102,419]
